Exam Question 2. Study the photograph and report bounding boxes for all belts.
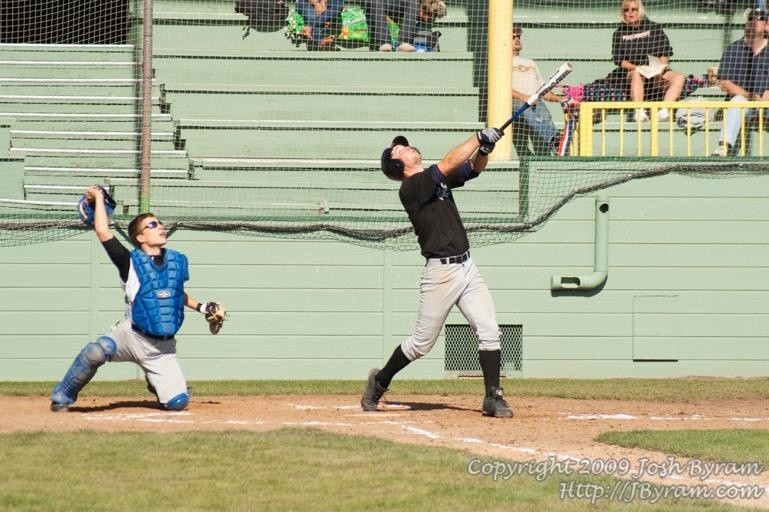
[440,250,470,264]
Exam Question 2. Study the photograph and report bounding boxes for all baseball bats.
[499,62,573,132]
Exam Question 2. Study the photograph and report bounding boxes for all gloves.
[477,127,505,156]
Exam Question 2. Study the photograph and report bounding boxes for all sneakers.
[51,401,68,411]
[557,125,568,157]
[482,385,513,417]
[632,107,646,122]
[361,369,388,410]
[658,109,669,122]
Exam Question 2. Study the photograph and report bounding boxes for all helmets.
[381,136,420,180]
[77,186,116,226]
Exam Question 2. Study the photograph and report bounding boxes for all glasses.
[138,221,163,234]
[624,7,637,13]
[747,16,764,22]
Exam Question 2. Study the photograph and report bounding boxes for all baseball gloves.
[206,302,226,334]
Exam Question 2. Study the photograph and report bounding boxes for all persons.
[611,0,684,122]
[366,0,421,52]
[361,135,515,419]
[51,186,219,414]
[710,5,769,158]
[512,27,572,157]
[293,0,346,49]
